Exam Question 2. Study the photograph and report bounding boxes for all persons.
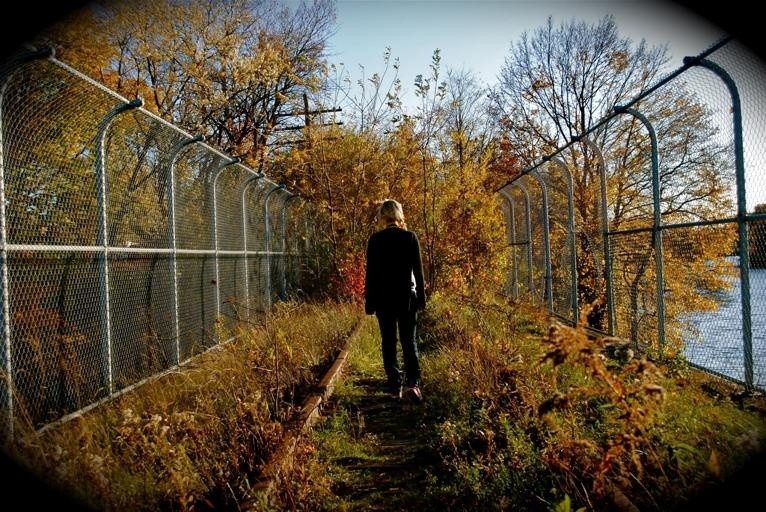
[363,198,428,401]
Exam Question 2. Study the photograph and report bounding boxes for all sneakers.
[405,385,424,403]
[391,385,403,400]
